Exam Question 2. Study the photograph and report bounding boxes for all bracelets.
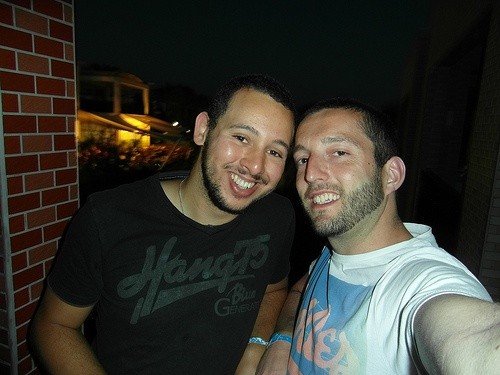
[248,334,269,347]
[268,332,292,347]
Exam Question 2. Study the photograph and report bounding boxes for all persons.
[29,76,291,374]
[250,96,500,375]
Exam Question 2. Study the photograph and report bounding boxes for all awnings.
[79,108,189,143]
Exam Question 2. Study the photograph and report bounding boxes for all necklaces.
[178,178,185,215]
